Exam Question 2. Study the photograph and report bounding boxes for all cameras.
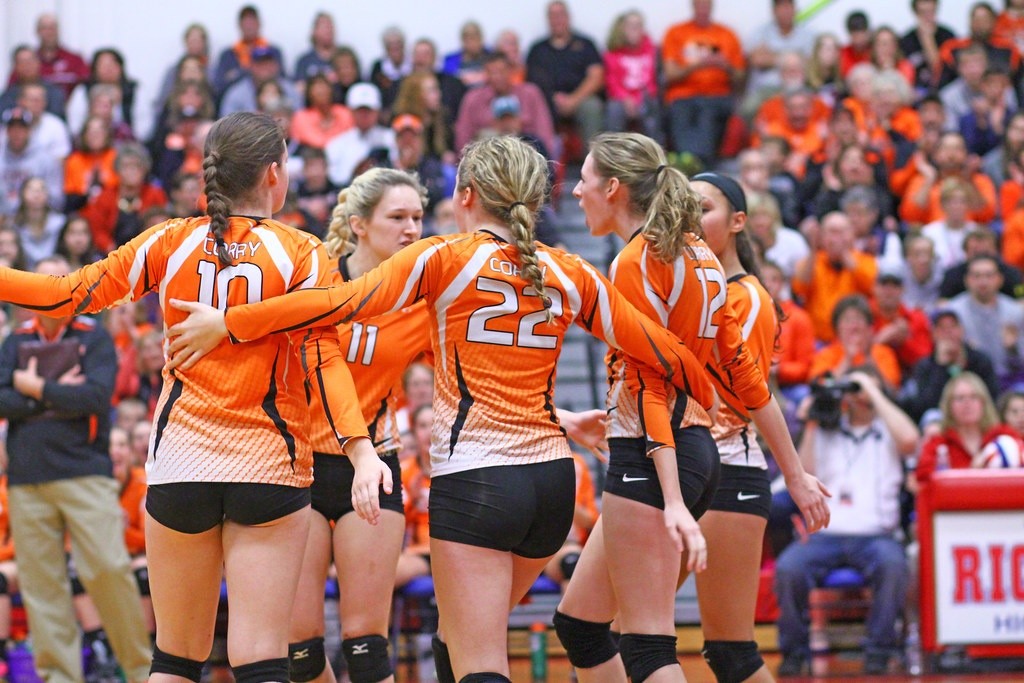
[812,375,843,429]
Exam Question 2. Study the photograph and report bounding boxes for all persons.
[0,254,154,682]
[0,0,1024,683]
[323,82,401,189]
[545,132,831,683]
[659,0,746,175]
[160,135,721,683]
[77,140,167,254]
[207,4,287,95]
[0,112,393,683]
[766,362,922,676]
[452,50,557,180]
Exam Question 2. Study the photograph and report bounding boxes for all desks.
[916,468,1024,659]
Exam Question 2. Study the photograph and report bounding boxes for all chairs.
[0,574,560,683]
[799,458,908,675]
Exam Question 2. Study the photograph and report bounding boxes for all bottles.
[935,444,951,471]
[907,621,922,675]
[528,620,548,682]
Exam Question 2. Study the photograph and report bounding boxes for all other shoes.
[776,648,811,675]
[863,653,888,672]
